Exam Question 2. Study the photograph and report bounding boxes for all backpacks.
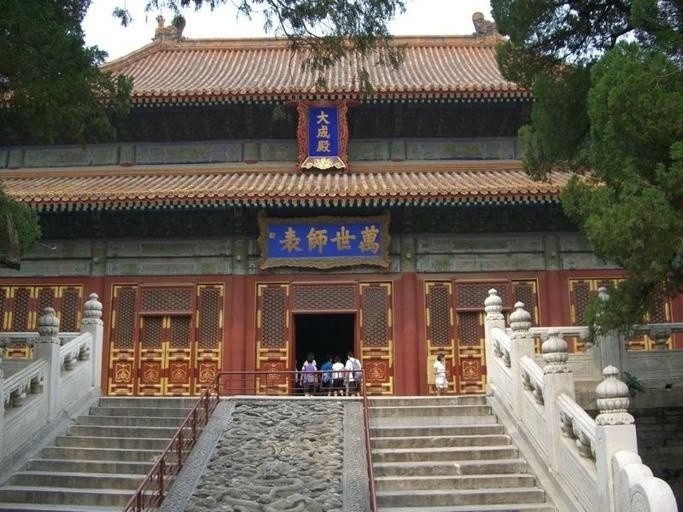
[350,359,362,379]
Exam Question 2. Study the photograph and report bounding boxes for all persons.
[295,350,362,397]
[433,355,449,396]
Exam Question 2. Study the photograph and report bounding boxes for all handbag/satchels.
[308,375,318,383]
